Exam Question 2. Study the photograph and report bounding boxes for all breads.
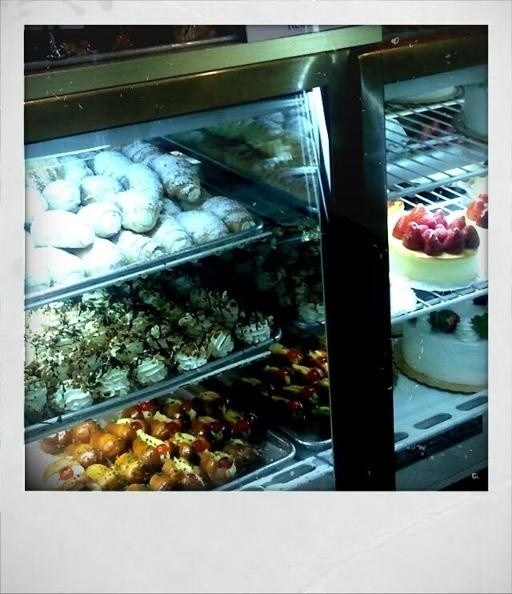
[25,105,319,292]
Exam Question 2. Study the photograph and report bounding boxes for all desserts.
[24,218,331,491]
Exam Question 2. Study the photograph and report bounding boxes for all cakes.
[386,176,487,393]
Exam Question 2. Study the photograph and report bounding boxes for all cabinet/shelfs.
[25,50,368,491]
[351,25,487,491]
